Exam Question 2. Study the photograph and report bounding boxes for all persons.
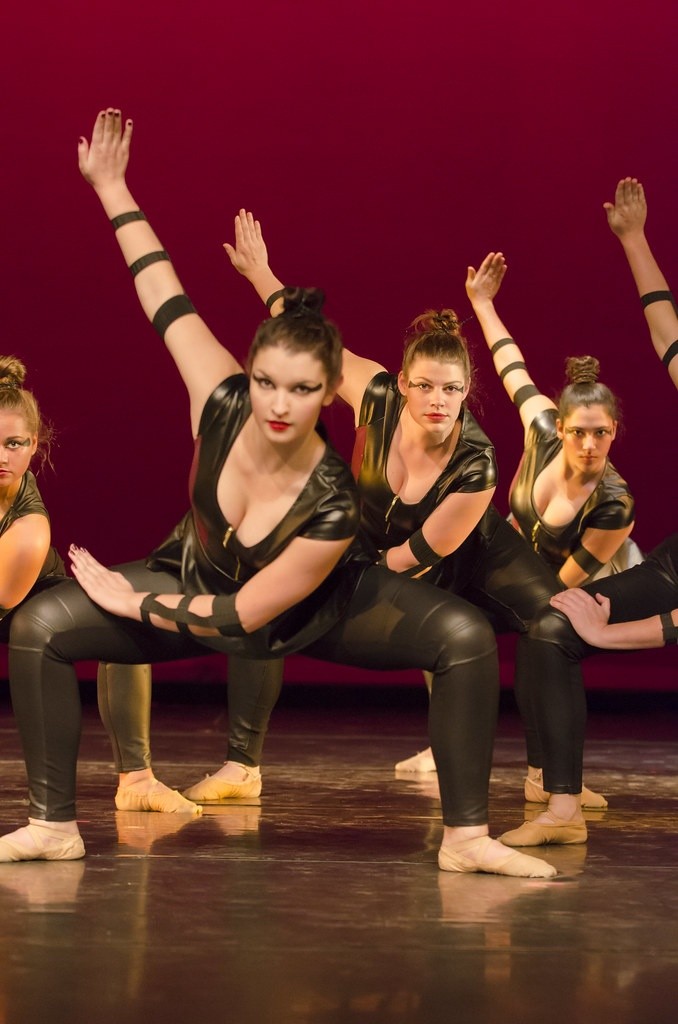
[1,108,558,879]
[0,356,202,813]
[395,253,678,771]
[500,178,678,847]
[182,209,608,807]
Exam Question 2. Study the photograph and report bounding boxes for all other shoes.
[525,770,608,808]
[0,860,85,895]
[115,779,200,813]
[395,750,437,772]
[0,824,86,863]
[438,870,548,910]
[511,844,587,871]
[524,802,607,822]
[182,761,262,800]
[438,835,558,879]
[192,797,261,828]
[115,810,202,839]
[499,809,587,846]
